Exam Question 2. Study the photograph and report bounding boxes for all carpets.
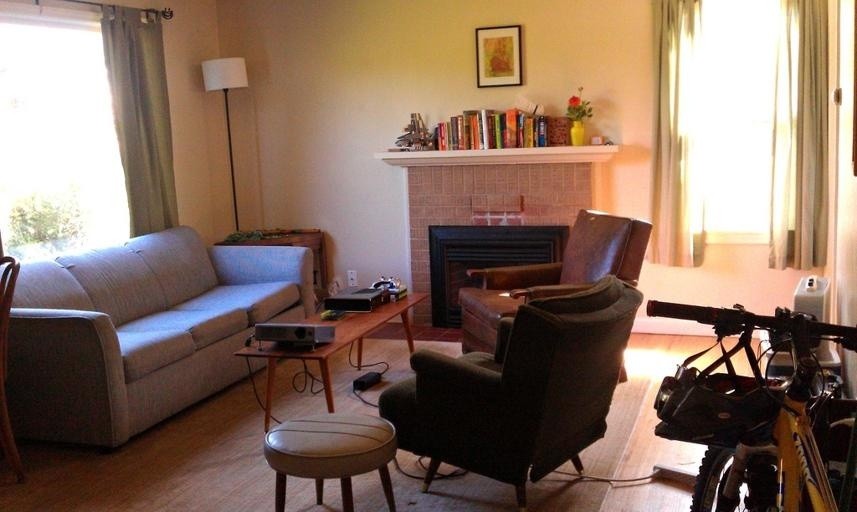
[0,331,651,512]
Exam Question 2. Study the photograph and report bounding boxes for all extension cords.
[652,462,698,487]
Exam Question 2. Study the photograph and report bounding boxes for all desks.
[220,284,422,412]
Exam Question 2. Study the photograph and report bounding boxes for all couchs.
[0,223,322,453]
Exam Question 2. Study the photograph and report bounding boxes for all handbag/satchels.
[652,306,782,450]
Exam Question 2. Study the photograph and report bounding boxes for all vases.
[568,120,587,146]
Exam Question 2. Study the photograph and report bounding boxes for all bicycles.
[646,299,857,512]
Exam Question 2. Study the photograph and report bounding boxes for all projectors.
[253,322,336,345]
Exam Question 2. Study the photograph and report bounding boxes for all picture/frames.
[474,25,523,89]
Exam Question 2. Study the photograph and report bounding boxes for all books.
[437,109,548,150]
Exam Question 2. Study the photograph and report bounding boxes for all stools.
[263,415,405,512]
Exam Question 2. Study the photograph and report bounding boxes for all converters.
[353,372,382,392]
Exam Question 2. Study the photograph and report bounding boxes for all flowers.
[564,87,593,122]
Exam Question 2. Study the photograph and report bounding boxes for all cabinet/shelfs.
[215,228,325,291]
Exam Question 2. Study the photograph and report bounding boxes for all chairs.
[447,213,655,386]
[1,256,29,483]
[377,274,643,451]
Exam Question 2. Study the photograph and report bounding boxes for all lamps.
[201,57,250,231]
